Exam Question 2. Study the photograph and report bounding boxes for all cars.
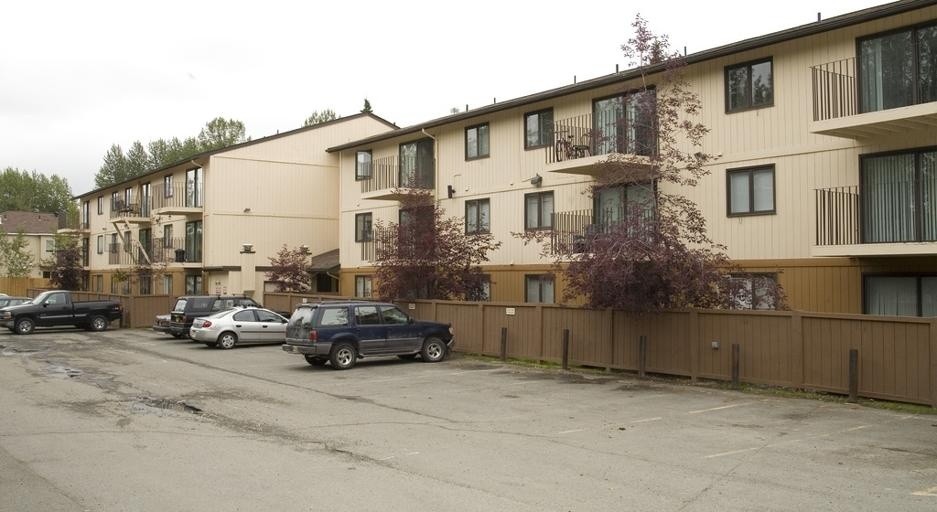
[151,314,171,331]
[189,306,289,349]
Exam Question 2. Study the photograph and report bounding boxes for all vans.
[171,296,263,339]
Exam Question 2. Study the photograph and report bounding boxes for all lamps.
[448,186,455,199]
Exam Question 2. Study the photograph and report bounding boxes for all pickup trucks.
[0,290,122,335]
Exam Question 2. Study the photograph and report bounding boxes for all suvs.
[283,301,454,371]
